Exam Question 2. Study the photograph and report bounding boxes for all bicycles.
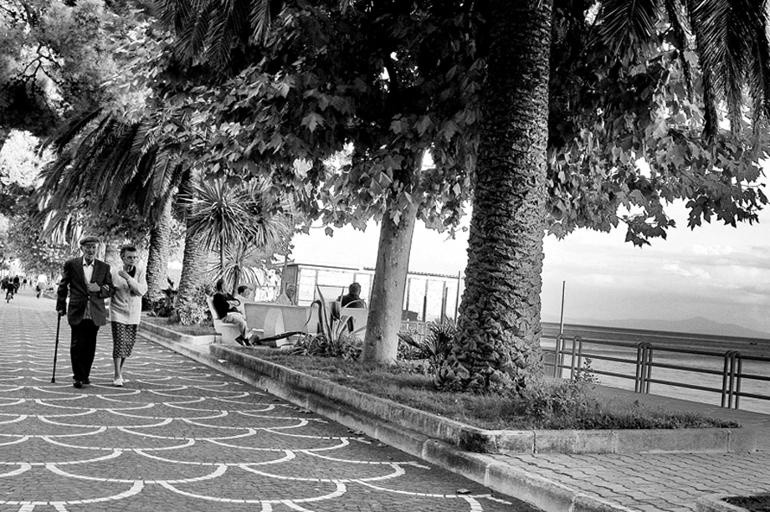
[4,283,18,304]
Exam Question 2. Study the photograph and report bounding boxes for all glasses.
[125,255,139,261]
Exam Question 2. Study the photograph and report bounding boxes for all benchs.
[205,294,369,345]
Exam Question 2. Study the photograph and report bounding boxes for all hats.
[80,236,100,245]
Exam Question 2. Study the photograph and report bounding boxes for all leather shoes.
[73,378,91,389]
[112,373,124,387]
[235,337,253,346]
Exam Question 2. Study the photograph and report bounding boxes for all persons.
[55,236,115,389]
[274,283,299,305]
[212,278,252,346]
[234,284,250,317]
[335,282,366,308]
[0,272,49,305]
[102,244,149,386]
